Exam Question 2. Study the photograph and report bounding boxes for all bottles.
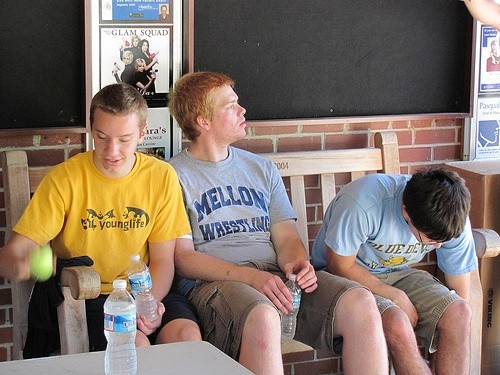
[104,280,137,375]
[127,254,158,322]
[279,274,301,341]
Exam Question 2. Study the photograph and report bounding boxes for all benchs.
[1,132,500,375]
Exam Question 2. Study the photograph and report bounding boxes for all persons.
[487,39,500,72]
[0,83,202,361]
[312,168,483,375]
[112,34,161,94]
[159,5,169,20]
[163,72,389,375]
[460,0,500,32]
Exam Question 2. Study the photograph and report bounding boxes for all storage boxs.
[431,158,500,375]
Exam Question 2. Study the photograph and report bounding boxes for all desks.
[0,340,256,375]
